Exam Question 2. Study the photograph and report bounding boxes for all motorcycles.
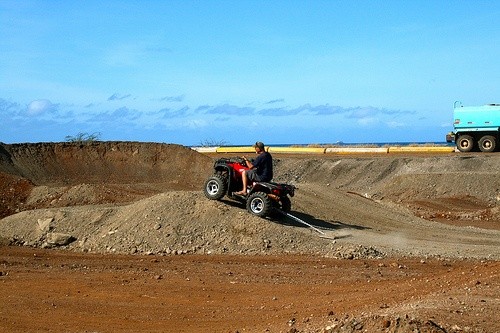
[204,157,295,221]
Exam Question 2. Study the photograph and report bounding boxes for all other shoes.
[236,190,248,196]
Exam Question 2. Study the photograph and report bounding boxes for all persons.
[234,141,273,195]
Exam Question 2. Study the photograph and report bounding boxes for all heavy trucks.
[444,99,500,153]
